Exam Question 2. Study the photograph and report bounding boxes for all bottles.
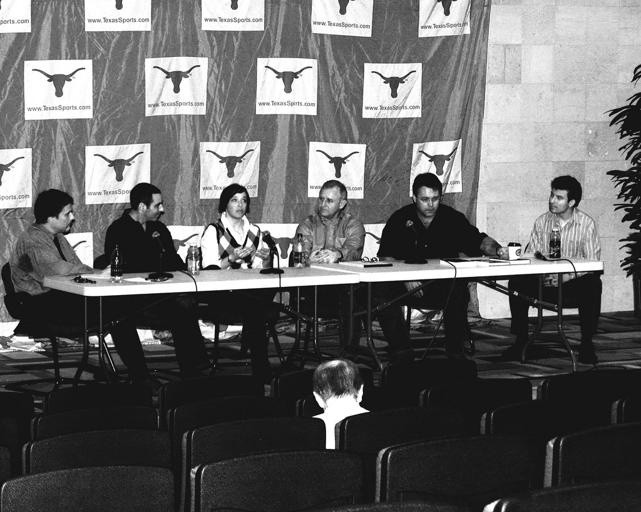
[111,242,123,283]
[187,242,201,275]
[294,232,306,268]
[548,226,561,258]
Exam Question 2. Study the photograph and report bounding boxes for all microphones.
[535,251,546,261]
[406,220,430,248]
[152,231,166,253]
[263,231,277,255]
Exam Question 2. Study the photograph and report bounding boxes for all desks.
[311,253,605,373]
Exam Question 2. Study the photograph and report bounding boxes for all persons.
[9,189,161,387]
[105,182,204,376]
[289,180,365,364]
[500,176,602,363]
[375,173,507,364]
[201,182,273,370]
[310,357,369,451]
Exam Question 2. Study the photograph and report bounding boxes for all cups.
[508,241,521,259]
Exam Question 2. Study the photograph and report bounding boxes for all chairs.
[0,363,641,512]
[2,259,109,373]
[92,257,192,363]
[189,254,289,367]
